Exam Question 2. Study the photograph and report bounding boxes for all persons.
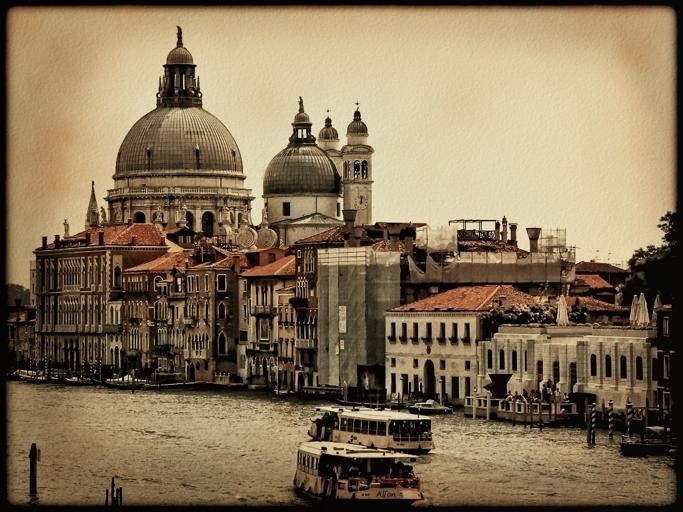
[505,379,570,402]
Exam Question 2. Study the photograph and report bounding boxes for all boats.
[291,436,424,504]
[410,398,454,419]
[305,399,437,456]
